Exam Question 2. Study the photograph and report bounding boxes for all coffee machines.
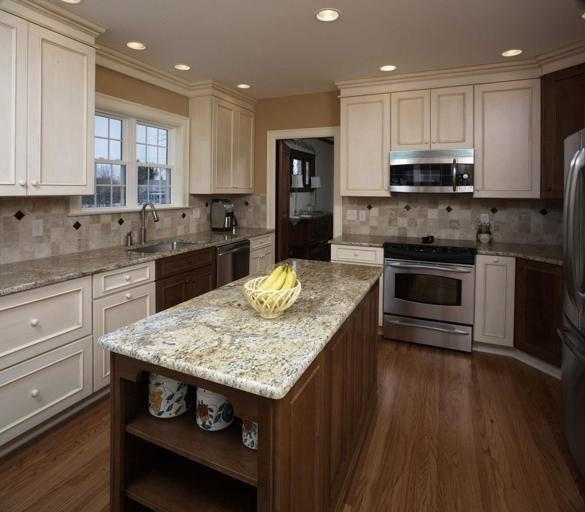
[209,198,235,231]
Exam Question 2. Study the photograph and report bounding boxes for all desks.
[289,210,331,259]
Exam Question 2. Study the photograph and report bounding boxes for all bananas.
[251,259,296,308]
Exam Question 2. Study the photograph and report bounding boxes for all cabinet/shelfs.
[188,96,256,195]
[390,85,474,150]
[541,62,585,200]
[339,93,392,198]
[0,9,95,198]
[156,246,217,313]
[0,275,93,447]
[330,245,384,327]
[472,254,516,348]
[474,77,541,199]
[109,351,274,511]
[93,259,157,394]
[514,257,564,368]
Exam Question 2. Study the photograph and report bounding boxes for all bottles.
[476,221,491,244]
[145,371,259,453]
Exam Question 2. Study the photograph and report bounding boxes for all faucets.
[140,202,160,245]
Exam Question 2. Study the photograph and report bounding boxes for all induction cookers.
[383,234,479,257]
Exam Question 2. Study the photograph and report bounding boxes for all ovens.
[382,261,475,355]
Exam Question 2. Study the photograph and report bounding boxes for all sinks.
[126,238,200,254]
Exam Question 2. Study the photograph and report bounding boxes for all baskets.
[244,275,301,319]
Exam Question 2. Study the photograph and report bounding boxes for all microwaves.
[389,148,475,195]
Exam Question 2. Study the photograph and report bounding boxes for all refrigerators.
[555,127,584,484]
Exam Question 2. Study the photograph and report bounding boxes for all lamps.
[291,174,304,216]
[311,176,321,212]
